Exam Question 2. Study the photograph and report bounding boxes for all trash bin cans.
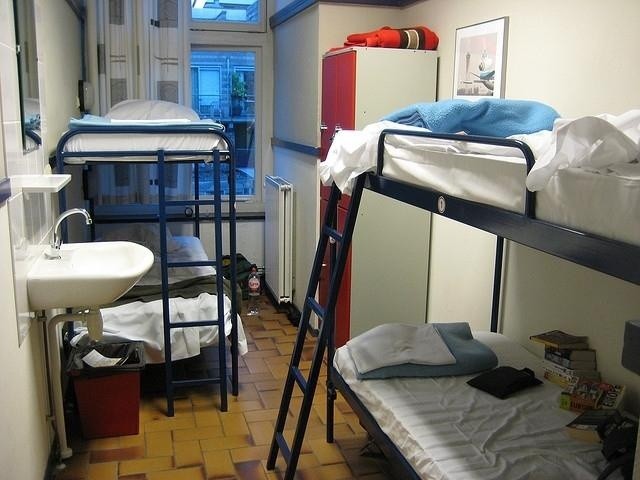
[65,341,145,440]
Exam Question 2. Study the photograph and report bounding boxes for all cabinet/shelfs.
[316,44,439,348]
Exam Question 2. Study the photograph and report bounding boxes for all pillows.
[104,95,201,122]
[101,221,178,254]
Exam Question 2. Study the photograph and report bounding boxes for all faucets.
[42,207,94,260]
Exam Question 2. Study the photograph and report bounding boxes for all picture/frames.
[450,14,509,104]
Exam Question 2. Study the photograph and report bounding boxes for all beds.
[321,124,639,479]
[56,111,243,397]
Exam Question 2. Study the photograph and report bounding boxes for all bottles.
[246,267,262,317]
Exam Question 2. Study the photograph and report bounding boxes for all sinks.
[28,240,155,312]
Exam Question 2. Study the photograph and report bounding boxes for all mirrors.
[14,0,45,155]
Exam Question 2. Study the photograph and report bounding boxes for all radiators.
[262,173,294,305]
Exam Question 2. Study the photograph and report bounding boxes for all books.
[530,329,640,444]
